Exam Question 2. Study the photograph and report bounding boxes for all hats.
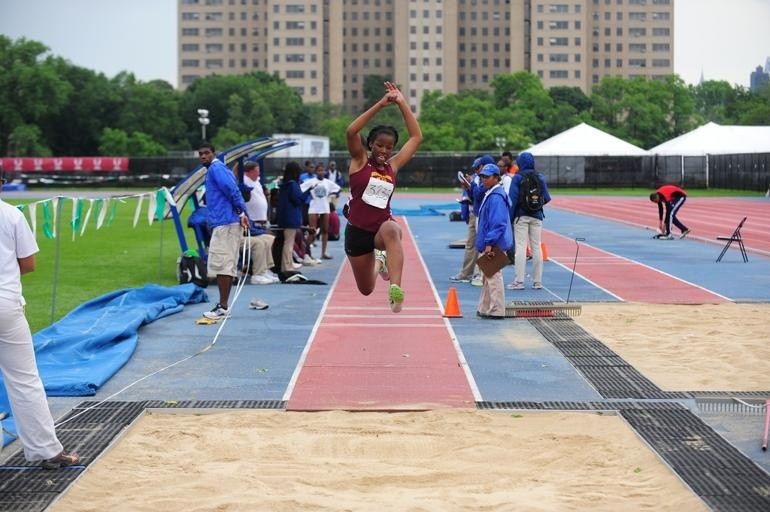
[239,184,254,192]
[479,154,495,166]
[478,164,499,177]
[470,157,483,167]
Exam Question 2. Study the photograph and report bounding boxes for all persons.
[233,161,270,228]
[506,152,551,290]
[342,80,423,313]
[262,187,315,265]
[449,157,482,283]
[187,186,279,284]
[299,160,317,231]
[270,189,321,265]
[327,202,340,241]
[502,151,519,175]
[300,165,342,259]
[0,196,79,471]
[650,185,691,240]
[463,155,494,285]
[276,161,319,272]
[474,164,514,319]
[198,142,250,320]
[324,160,344,207]
[496,159,512,196]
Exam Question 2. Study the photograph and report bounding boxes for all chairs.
[716,216,748,264]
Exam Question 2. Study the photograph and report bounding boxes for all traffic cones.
[540,241,548,261]
[442,286,463,318]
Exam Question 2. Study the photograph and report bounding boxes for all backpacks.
[514,170,545,224]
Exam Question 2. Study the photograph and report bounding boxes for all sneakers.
[532,281,543,290]
[479,314,506,319]
[389,285,406,313]
[44,454,82,470]
[448,274,472,283]
[660,234,674,240]
[250,275,272,285]
[471,276,484,288]
[375,250,391,283]
[202,304,233,321]
[264,275,280,284]
[679,229,693,239]
[507,281,526,290]
[247,298,270,311]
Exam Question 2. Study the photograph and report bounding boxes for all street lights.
[198,108,210,139]
